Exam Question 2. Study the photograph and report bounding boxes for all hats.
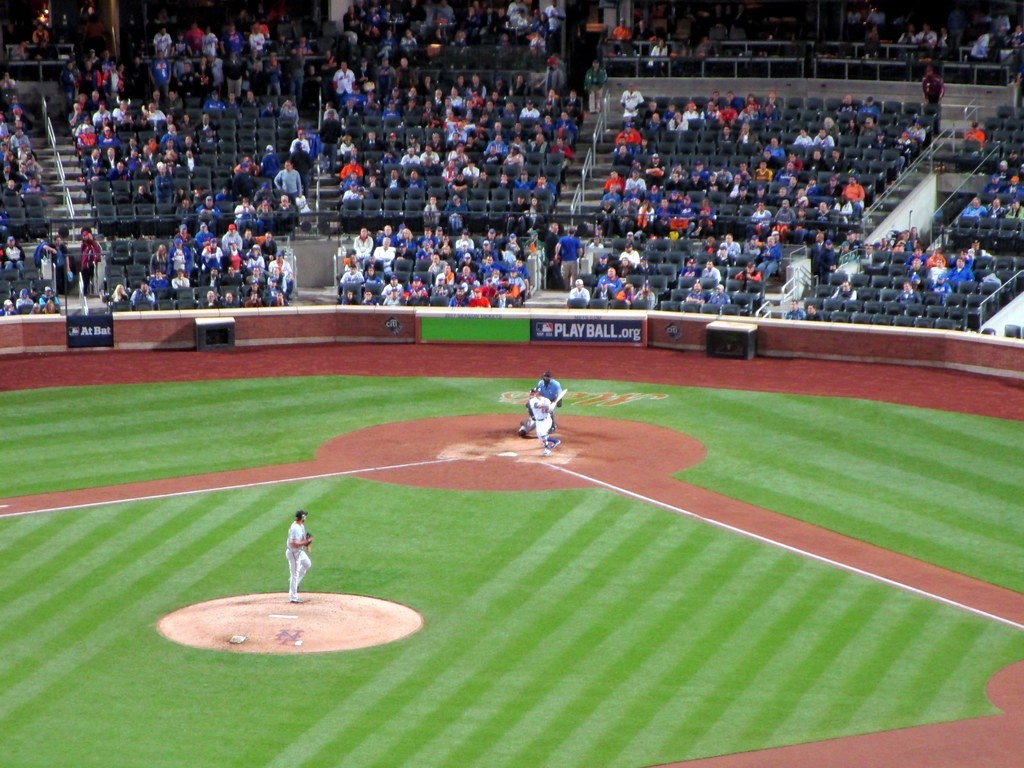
[544,371,552,378]
[296,511,308,519]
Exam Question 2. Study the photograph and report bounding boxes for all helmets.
[533,387,542,395]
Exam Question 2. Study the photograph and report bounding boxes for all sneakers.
[551,440,561,449]
[543,450,552,457]
[291,598,304,604]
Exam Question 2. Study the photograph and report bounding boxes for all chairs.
[0,92,1024,335]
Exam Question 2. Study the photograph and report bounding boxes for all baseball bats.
[549,388,567,413]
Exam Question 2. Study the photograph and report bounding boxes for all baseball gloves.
[306,532,313,548]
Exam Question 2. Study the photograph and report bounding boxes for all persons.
[530,386,561,456]
[0,0,1024,321]
[285,510,313,603]
[536,370,562,432]
